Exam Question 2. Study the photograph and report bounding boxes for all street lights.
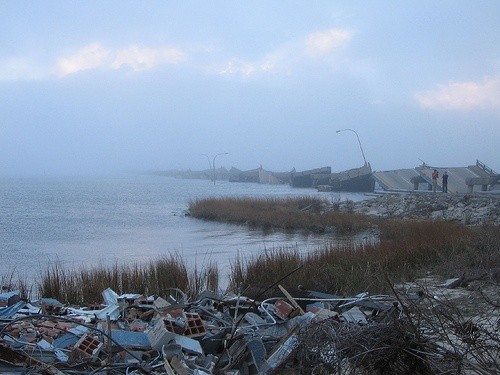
[336,128,366,165]
[214,153,228,181]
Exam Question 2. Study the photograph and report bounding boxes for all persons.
[441,170,450,194]
[430,169,440,193]
